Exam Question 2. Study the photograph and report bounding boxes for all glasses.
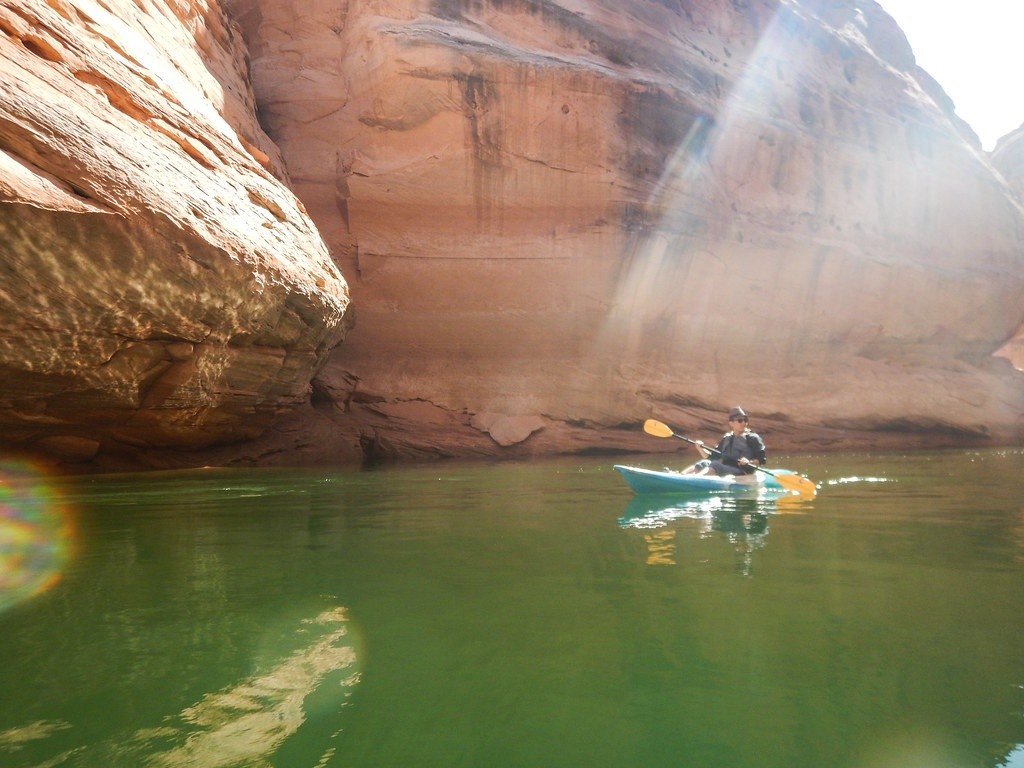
[732,419,748,423]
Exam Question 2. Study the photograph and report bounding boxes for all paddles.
[644,418,816,495]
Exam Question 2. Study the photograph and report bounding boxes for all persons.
[663,407,766,477]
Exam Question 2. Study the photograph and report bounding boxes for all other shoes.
[663,466,670,474]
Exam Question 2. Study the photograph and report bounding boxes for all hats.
[729,406,747,420]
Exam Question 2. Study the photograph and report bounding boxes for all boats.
[613,464,795,495]
